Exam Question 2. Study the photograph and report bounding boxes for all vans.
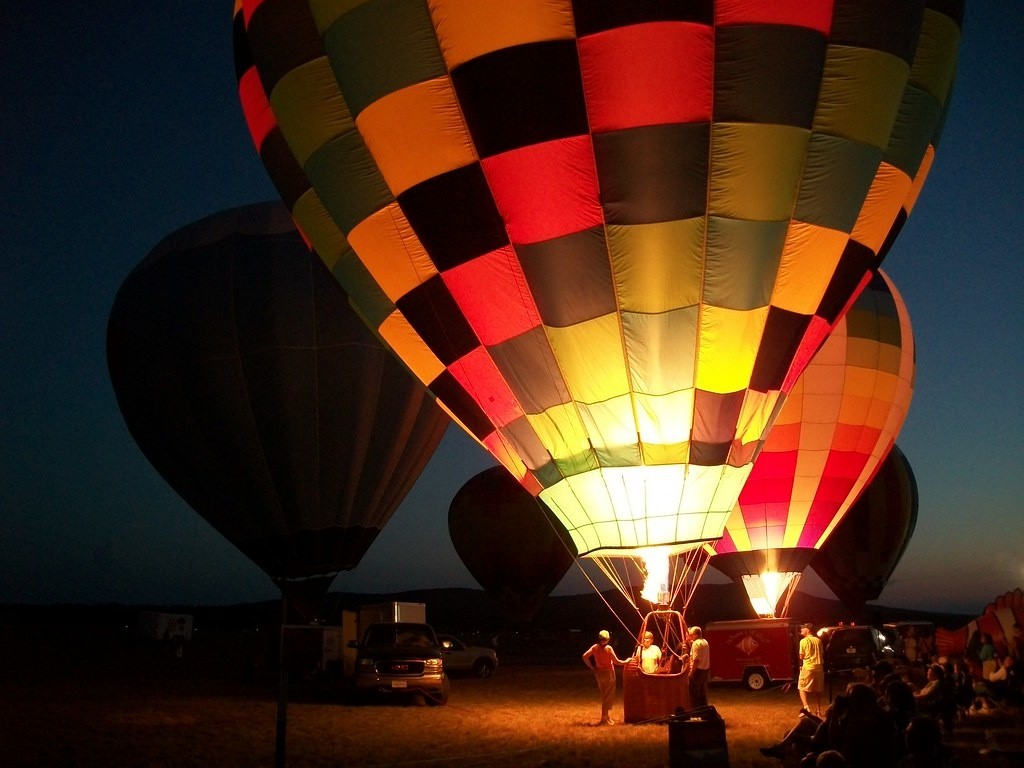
[346,621,451,706]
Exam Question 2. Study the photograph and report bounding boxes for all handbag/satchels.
[989,666,1006,681]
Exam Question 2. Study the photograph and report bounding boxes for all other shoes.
[799,708,810,718]
[965,708,977,716]
[600,719,614,725]
[976,707,991,714]
[759,742,786,759]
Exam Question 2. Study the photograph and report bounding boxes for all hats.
[871,660,891,674]
[801,622,815,632]
[926,663,944,678]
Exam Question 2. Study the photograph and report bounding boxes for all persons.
[757,617,1024,768]
[632,631,662,675]
[668,624,709,711]
[343,638,357,674]
[582,630,635,727]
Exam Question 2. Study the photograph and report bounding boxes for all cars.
[434,634,498,680]
[815,625,880,677]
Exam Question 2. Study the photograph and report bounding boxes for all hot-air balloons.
[107,200,452,697]
[448,466,580,634]
[715,268,916,621]
[228,0,960,726]
[809,444,919,623]
